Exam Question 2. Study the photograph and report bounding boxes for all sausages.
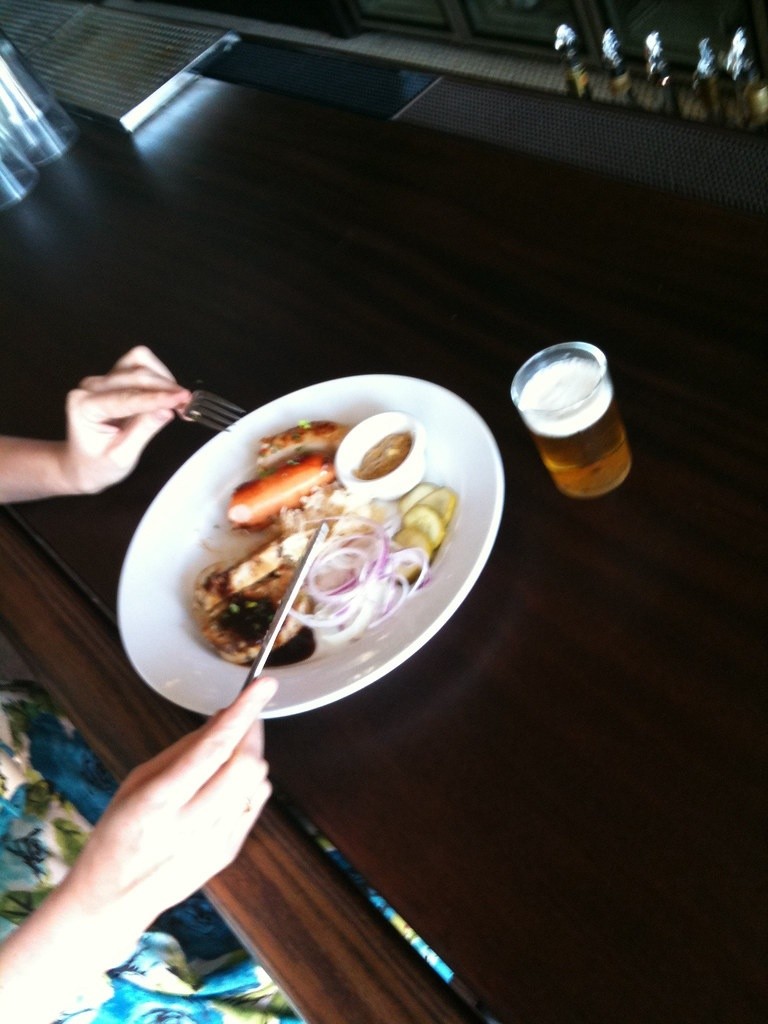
[226,420,351,521]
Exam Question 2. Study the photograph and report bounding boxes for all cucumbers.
[391,482,458,582]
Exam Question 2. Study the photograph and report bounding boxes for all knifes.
[236,521,329,694]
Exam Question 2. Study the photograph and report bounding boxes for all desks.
[0,34,768,1024]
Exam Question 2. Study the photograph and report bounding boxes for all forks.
[173,389,244,434]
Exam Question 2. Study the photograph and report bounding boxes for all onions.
[289,514,427,640]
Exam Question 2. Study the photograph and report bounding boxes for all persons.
[0,344,454,1024]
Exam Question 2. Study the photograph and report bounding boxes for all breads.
[194,537,308,664]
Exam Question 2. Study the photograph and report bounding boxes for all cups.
[509,340,632,499]
[0,27,80,165]
[0,116,39,212]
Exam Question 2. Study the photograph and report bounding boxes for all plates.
[117,372,506,723]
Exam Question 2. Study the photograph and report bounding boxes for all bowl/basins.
[332,410,428,501]
